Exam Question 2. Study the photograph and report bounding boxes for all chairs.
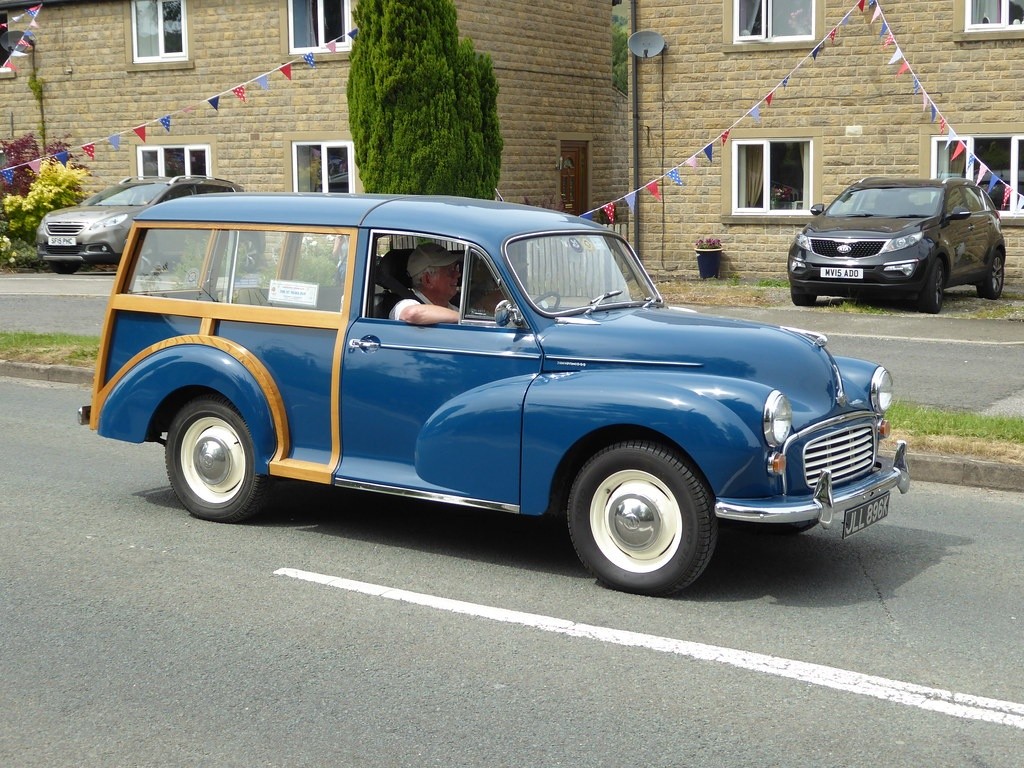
[370,248,416,320]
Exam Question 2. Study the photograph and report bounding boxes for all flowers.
[696,238,722,250]
[772,185,793,201]
[788,9,811,28]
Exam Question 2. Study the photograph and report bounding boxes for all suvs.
[785,173,1008,317]
[77,193,913,598]
[32,175,266,274]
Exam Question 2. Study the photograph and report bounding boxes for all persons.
[332,234,350,286]
[389,242,461,324]
[471,258,530,317]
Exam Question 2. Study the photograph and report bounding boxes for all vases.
[695,249,721,279]
[796,28,809,35]
[773,199,784,209]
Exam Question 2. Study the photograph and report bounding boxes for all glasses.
[434,263,460,276]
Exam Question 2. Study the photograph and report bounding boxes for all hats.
[407,243,463,277]
[475,260,529,280]
